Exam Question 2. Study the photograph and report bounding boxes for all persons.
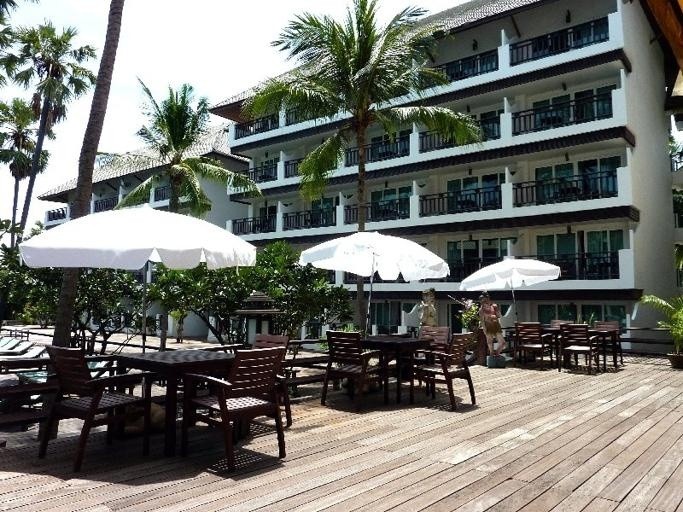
[478,292,507,360]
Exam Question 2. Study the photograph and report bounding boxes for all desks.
[114,348,237,459]
[499,325,620,371]
[360,335,434,404]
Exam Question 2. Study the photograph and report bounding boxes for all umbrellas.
[459,256,560,362]
[16,201,257,437]
[297,229,452,397]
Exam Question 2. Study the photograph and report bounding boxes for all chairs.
[513,321,552,369]
[183,346,290,473]
[551,319,575,359]
[320,329,381,410]
[249,334,294,427]
[557,322,600,373]
[405,332,477,410]
[399,324,450,397]
[594,320,624,368]
[37,343,148,475]
